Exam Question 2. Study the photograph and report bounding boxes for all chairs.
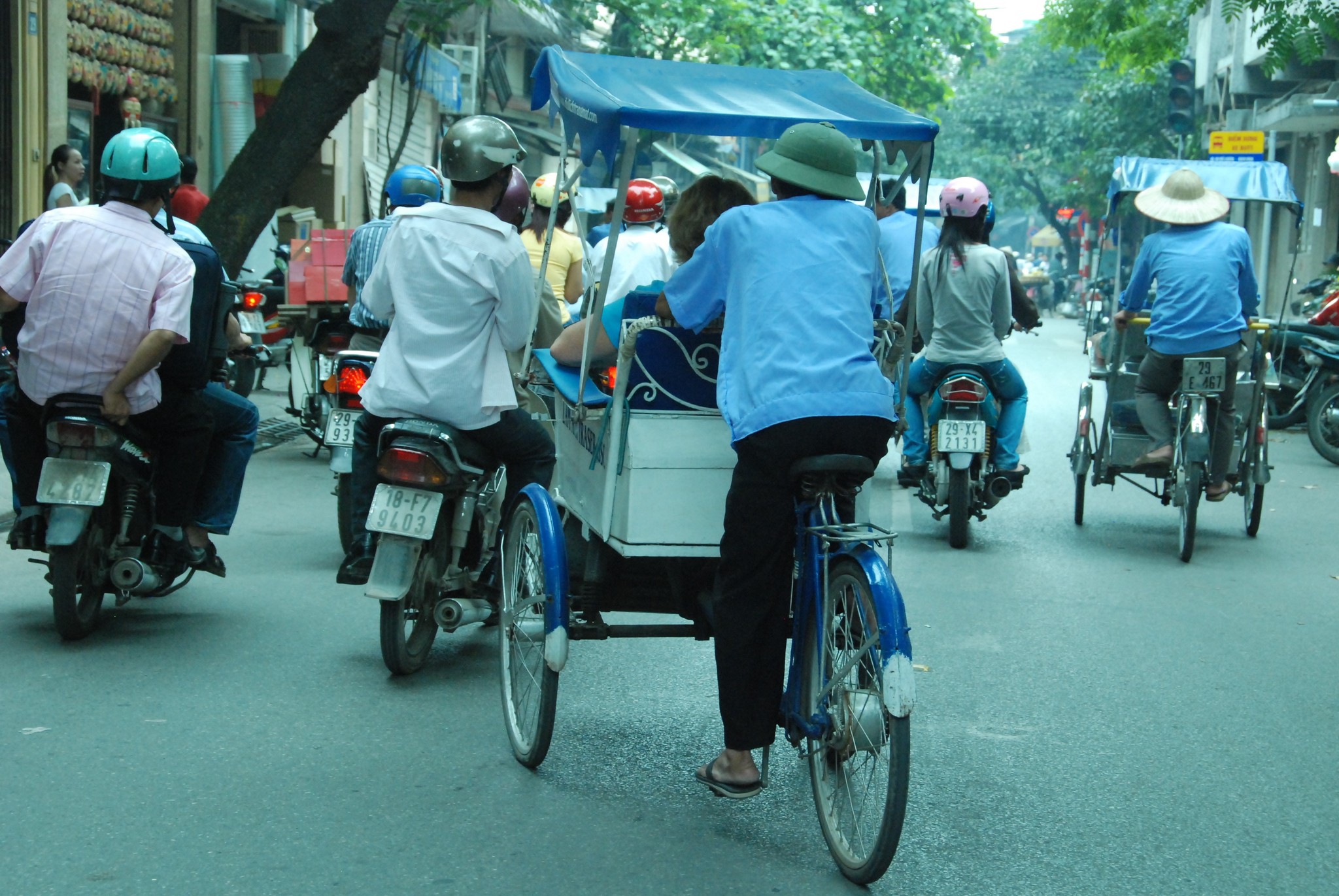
[613,287,725,413]
[1097,313,1263,378]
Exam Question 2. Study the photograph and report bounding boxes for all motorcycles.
[341,414,509,677]
[5,289,272,641]
[896,319,1044,550]
[1252,254,1339,464]
[207,259,388,551]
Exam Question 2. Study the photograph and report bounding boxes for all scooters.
[1077,275,1113,337]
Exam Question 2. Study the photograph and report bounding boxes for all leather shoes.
[139,529,207,564]
[337,533,378,585]
[8,514,46,550]
[477,556,503,591]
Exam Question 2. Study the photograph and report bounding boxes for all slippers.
[188,539,225,577]
[694,757,762,798]
[1131,453,1172,469]
[1205,480,1232,501]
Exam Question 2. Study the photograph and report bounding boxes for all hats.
[1134,169,1230,225]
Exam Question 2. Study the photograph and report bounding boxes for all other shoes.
[996,464,1029,480]
[901,463,929,478]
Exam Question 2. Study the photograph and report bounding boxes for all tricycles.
[1065,155,1306,562]
[496,43,941,886]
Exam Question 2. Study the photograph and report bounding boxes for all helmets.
[384,165,441,206]
[622,178,665,223]
[490,165,530,227]
[100,127,181,180]
[648,176,680,208]
[985,201,995,223]
[530,172,578,207]
[440,115,528,182]
[939,176,989,217]
[424,166,443,199]
[754,123,866,201]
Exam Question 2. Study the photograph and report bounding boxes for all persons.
[657,126,899,799]
[0,113,1066,585]
[1107,163,1261,503]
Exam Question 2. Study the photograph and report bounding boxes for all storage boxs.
[276,222,358,310]
